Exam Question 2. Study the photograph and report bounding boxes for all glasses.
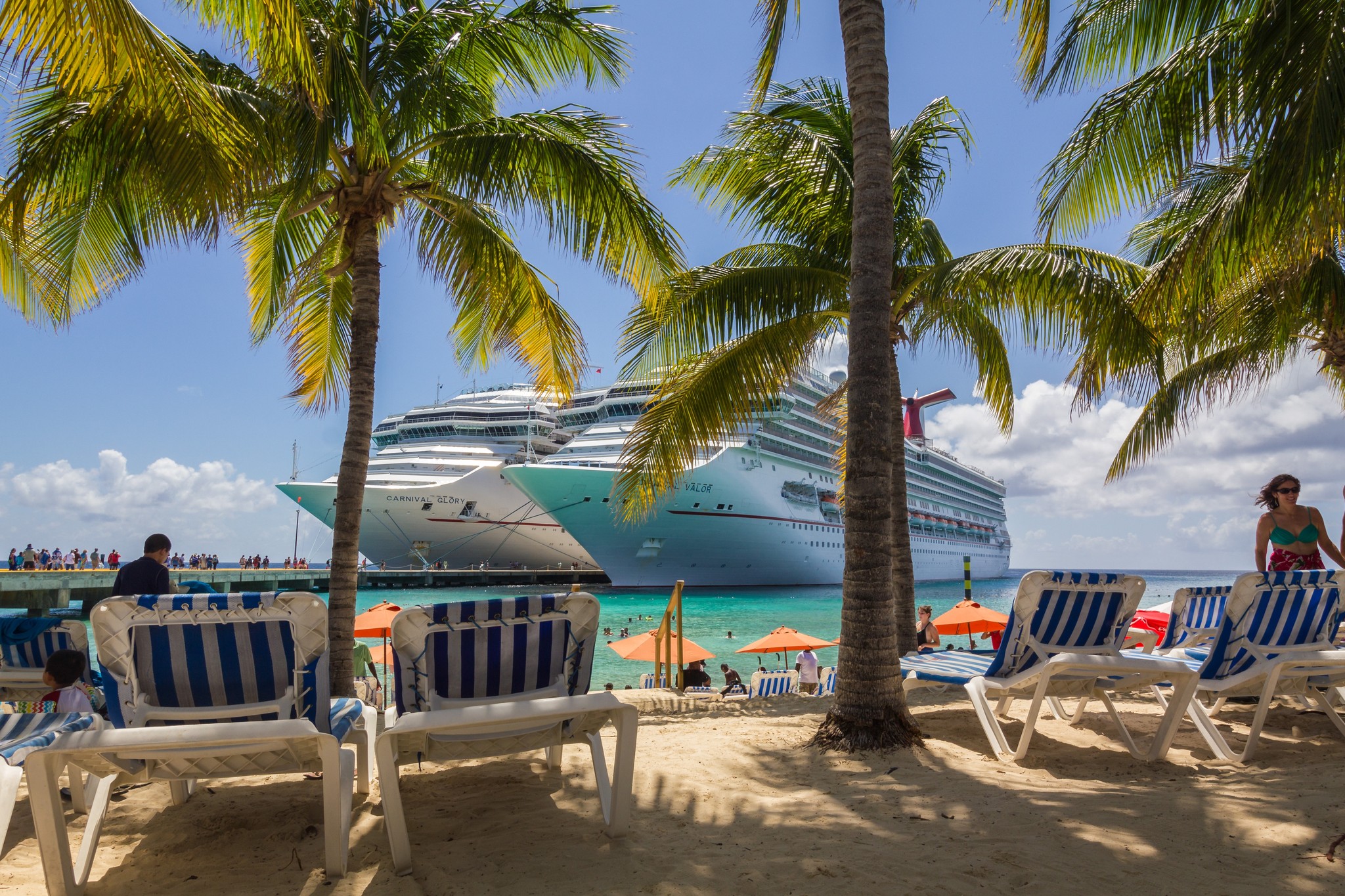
[1275,487,1300,494]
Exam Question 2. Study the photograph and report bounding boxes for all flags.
[596,369,601,373]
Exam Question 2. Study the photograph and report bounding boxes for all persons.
[558,562,578,570]
[381,560,385,571]
[42,650,128,797]
[1255,473,1345,572]
[604,615,651,644]
[8,544,121,571]
[728,631,732,636]
[327,558,332,569]
[795,649,823,694]
[353,637,382,690]
[480,560,488,571]
[509,561,518,570]
[240,554,307,569]
[672,616,675,620]
[437,560,448,571]
[676,659,766,691]
[112,533,171,596]
[363,557,366,570]
[164,552,218,570]
[916,605,1004,650]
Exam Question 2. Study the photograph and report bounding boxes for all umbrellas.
[608,628,716,673]
[734,625,840,670]
[353,599,404,710]
[930,598,1009,650]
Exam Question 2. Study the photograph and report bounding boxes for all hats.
[71,549,76,551]
[114,551,119,554]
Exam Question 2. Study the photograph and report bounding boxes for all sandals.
[304,771,323,778]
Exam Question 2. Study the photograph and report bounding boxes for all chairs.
[683,665,837,702]
[639,671,673,689]
[0,589,642,896]
[898,567,1345,764]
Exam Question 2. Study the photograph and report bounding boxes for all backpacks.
[326,560,330,564]
[51,552,59,560]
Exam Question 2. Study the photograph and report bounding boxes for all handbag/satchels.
[63,554,67,561]
[99,562,105,569]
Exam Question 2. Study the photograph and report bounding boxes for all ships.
[275,382,610,575]
[501,354,1014,586]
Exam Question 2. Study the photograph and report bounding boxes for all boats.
[946,520,958,531]
[934,519,948,529]
[820,494,841,513]
[956,521,970,534]
[910,513,926,525]
[977,526,985,536]
[968,525,978,534]
[923,515,937,528]
[908,512,912,522]
[984,528,993,537]
